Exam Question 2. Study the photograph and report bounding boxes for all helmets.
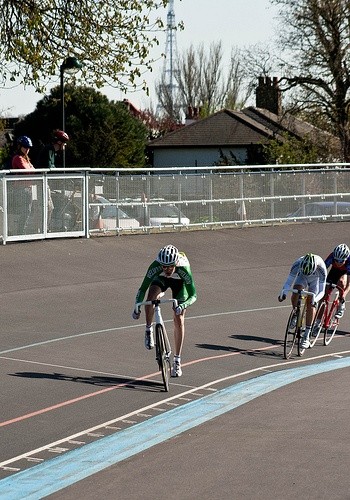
[48,129,69,142]
[300,253,317,276]
[17,136,33,148]
[333,243,350,260]
[157,244,179,266]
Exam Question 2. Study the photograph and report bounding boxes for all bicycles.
[309,283,344,348]
[280,288,316,360]
[135,298,179,393]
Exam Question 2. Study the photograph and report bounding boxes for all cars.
[287,202,350,218]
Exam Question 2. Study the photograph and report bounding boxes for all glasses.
[162,265,175,268]
[335,259,344,263]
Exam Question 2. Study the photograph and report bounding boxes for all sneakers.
[144,331,154,350]
[172,360,183,377]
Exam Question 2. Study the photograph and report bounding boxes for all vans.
[7,191,139,237]
[108,198,190,226]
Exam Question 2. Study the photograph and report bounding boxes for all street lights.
[59,57,82,231]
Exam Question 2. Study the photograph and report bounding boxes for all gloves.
[132,308,142,319]
[172,305,183,316]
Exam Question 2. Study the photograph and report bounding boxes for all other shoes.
[290,317,297,328]
[334,309,345,319]
[301,339,310,348]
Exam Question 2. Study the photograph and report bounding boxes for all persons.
[11,136,37,234]
[132,245,198,377]
[35,129,69,233]
[313,243,350,330]
[277,253,327,349]
